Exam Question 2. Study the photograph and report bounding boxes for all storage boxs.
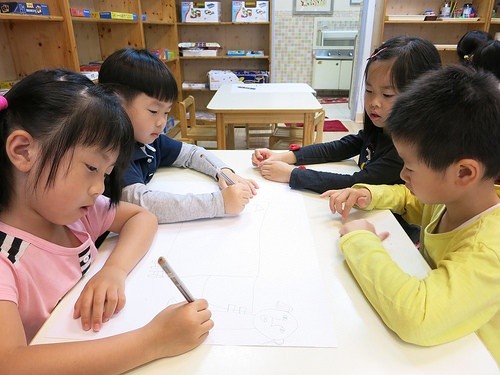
[0,2,51,14]
[70,7,137,20]
[178,42,269,92]
[230,0,270,22]
[165,118,175,134]
[150,47,174,61]
[181,1,222,23]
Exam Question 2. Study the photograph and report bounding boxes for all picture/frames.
[293,0,335,15]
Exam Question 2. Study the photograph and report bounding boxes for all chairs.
[245,109,326,152]
[179,94,230,150]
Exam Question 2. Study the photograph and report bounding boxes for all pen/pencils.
[158,257,195,302]
[200,154,235,185]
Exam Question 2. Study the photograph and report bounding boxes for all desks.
[27,148,500,375]
[207,84,323,148]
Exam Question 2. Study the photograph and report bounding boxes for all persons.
[320,64,500,367]
[95,47,260,249]
[252,36,442,242]
[0,69,214,375]
[457,30,500,81]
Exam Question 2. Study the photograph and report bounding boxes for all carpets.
[316,96,349,105]
[284,120,350,132]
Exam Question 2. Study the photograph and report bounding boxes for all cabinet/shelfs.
[0,0,182,148]
[176,1,272,126]
[314,60,352,90]
[381,0,500,60]
